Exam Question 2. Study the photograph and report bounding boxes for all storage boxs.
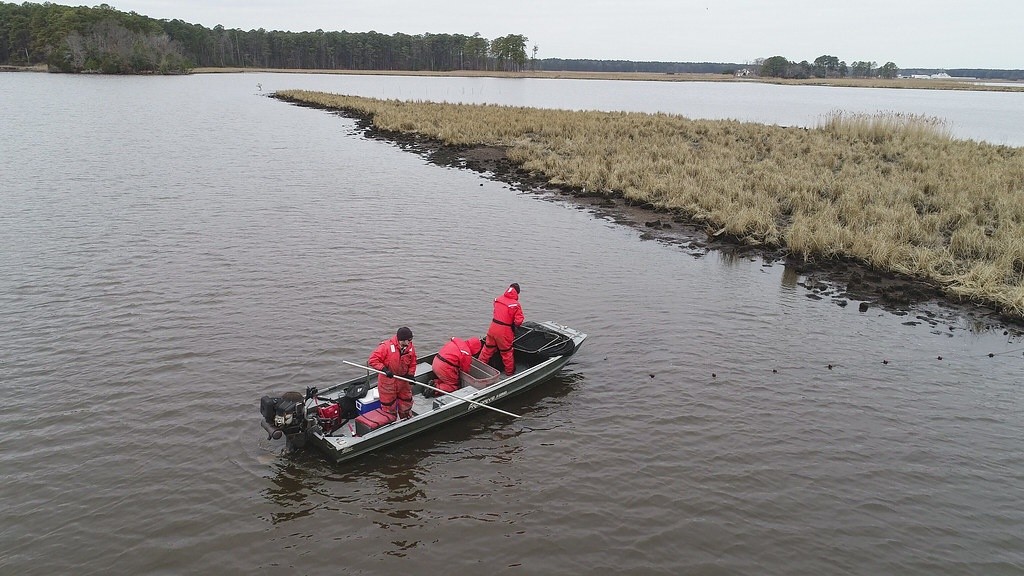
[459,356,501,390]
[486,326,560,363]
[355,386,398,416]
[355,408,397,437]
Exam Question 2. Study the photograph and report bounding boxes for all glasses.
[407,337,412,341]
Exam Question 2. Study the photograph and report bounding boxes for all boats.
[260,318,587,465]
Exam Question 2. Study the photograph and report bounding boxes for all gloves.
[407,375,417,385]
[381,366,393,378]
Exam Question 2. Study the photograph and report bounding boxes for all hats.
[396,327,413,341]
[510,283,520,294]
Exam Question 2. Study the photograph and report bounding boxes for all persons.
[478,283,525,376]
[421,336,482,399]
[368,327,417,419]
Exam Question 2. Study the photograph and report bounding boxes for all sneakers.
[422,380,435,398]
